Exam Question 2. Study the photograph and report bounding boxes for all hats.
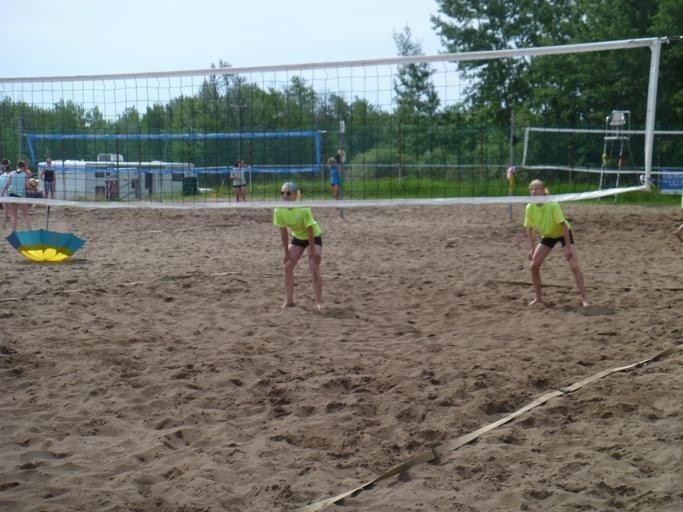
[29,178,39,186]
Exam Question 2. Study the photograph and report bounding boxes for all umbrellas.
[5,206,86,263]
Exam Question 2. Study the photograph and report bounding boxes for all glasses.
[280,191,291,195]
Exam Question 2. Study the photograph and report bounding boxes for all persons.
[230,160,250,202]
[327,157,342,199]
[524,179,591,311]
[273,182,328,311]
[0,158,56,232]
